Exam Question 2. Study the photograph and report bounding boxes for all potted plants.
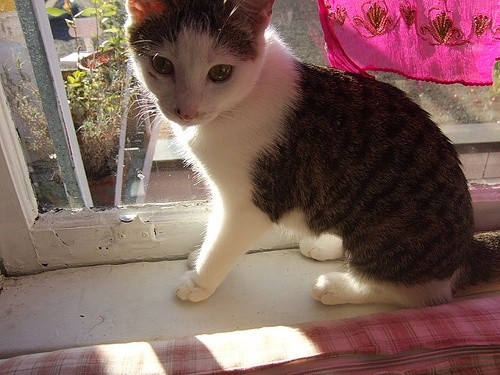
[43,1,128,71]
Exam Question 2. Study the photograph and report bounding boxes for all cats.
[122,1,500,309]
